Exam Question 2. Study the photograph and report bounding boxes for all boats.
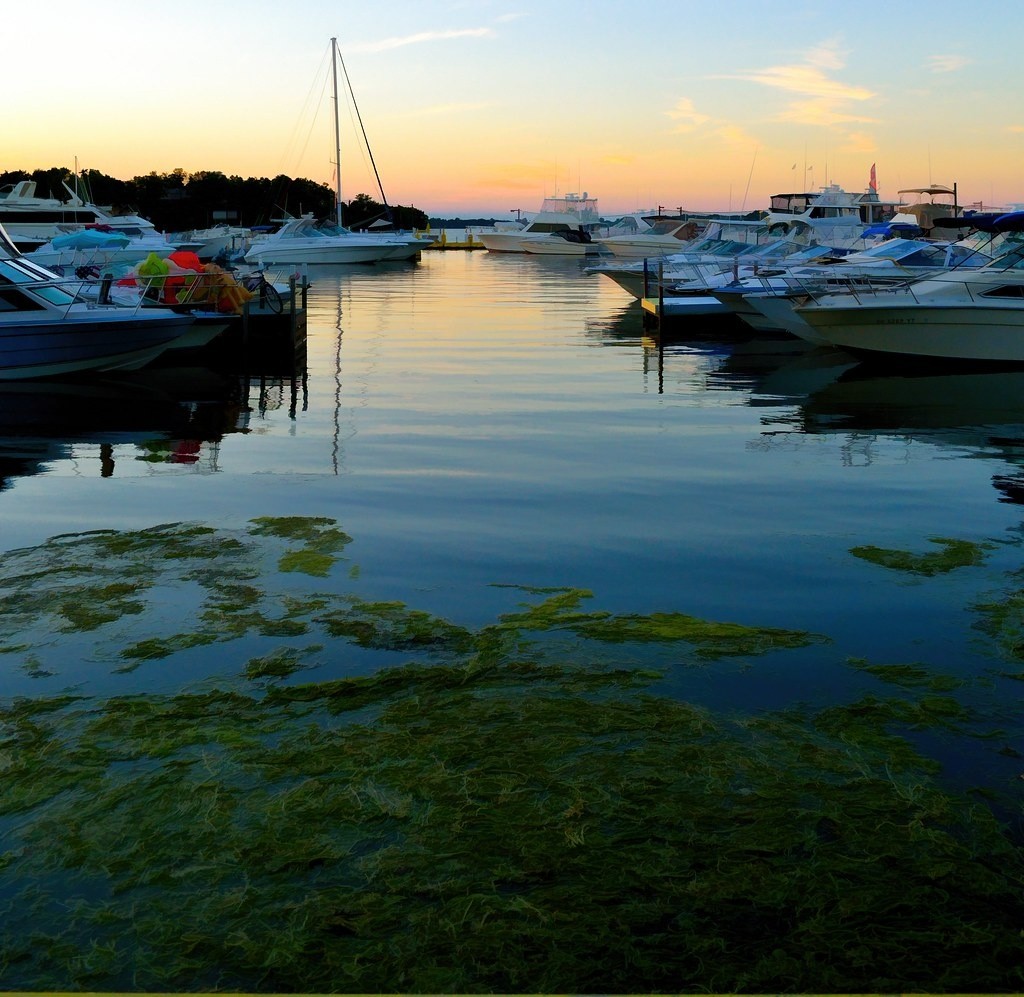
[0,36,1023,377]
